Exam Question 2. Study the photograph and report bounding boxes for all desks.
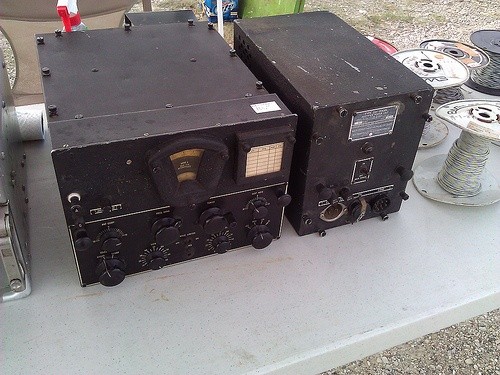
[0,94,500,375]
